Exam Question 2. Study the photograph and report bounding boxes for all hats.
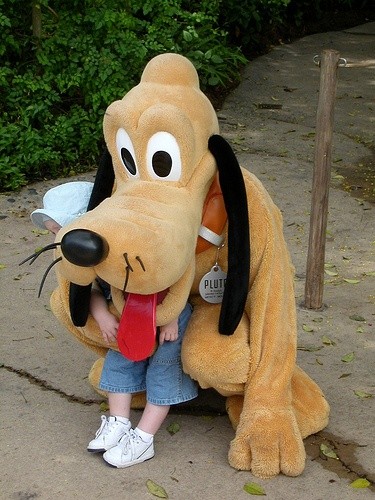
[30,181,94,228]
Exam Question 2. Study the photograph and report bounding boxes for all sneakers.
[102,429,155,469]
[85,416,131,452]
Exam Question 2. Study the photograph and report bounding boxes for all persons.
[29,181,201,467]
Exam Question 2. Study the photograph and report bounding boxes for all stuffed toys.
[14,51,329,477]
